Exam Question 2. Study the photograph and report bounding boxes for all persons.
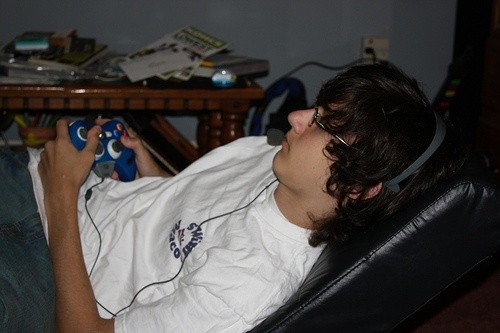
[0,61,458,333]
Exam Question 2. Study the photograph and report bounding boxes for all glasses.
[308,103,351,147]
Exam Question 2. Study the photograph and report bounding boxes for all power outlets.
[363,38,389,63]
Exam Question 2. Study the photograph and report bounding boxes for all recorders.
[14,31,54,50]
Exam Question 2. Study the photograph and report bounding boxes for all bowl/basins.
[19,126,54,144]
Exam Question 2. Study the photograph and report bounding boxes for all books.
[0,24,245,86]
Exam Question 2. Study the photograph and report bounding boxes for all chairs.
[245,121,499,333]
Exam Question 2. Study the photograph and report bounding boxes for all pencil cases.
[15,31,51,51]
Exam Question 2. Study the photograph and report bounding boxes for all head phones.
[329,112,446,192]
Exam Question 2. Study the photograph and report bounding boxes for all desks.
[0,77,264,170]
